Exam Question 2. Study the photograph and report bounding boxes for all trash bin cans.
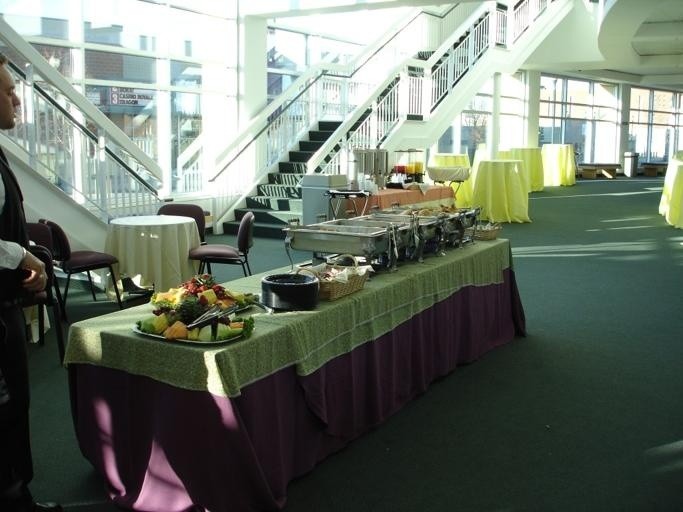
[302,174,350,226]
[623,151,639,178]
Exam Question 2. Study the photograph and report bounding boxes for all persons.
[0,54,60,512]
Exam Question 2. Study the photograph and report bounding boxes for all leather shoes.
[29,500,63,512]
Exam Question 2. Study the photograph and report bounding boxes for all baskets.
[297,254,368,302]
[463,223,504,240]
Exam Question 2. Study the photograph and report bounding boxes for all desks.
[345,186,457,215]
[325,190,372,220]
[432,144,576,224]
[63,238,525,512]
[658,151,683,228]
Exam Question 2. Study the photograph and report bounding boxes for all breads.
[402,204,458,215]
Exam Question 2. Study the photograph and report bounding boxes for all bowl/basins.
[259,273,318,311]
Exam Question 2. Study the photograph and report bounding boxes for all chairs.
[23,204,255,366]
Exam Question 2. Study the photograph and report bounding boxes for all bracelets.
[22,246,26,262]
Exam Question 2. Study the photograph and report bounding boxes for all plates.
[131,325,243,345]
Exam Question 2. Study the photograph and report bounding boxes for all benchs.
[579,164,621,180]
[641,162,667,176]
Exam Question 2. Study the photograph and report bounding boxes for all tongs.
[185,302,238,332]
[247,297,274,316]
[386,222,399,260]
[473,205,482,237]
[433,212,449,257]
[455,209,469,248]
[409,219,425,264]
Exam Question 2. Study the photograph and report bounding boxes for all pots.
[351,148,389,190]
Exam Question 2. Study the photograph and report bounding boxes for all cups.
[357,177,378,194]
